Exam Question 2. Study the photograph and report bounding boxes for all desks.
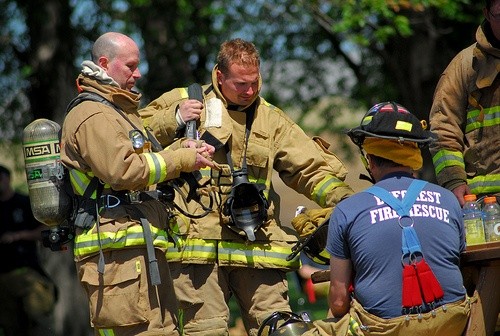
[312,242,500,336]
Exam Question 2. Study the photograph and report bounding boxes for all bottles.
[462,194,486,245]
[482,196,500,242]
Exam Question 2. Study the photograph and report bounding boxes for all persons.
[0,166,59,303]
[429,0,500,336]
[300,101,471,336]
[138,37,353,336]
[60,32,215,336]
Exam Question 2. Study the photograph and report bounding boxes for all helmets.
[286,208,330,265]
[345,101,437,144]
